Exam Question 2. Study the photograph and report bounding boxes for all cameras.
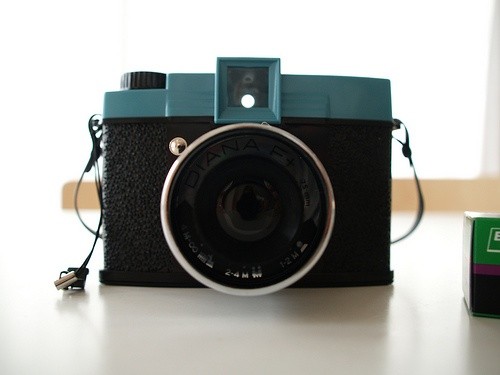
[99,56,395,297]
